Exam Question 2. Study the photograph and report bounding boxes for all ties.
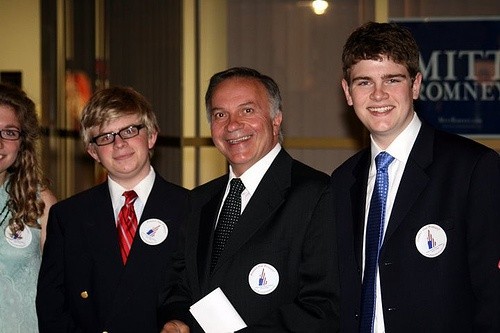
[209,178,246,278]
[116,190,139,267]
[358,152,395,333]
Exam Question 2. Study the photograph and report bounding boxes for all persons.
[161,67,362,333]
[0,88,60,333]
[36,88,198,331]
[326,19,499,333]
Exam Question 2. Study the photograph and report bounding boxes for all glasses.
[0,129,23,141]
[89,123,146,147]
